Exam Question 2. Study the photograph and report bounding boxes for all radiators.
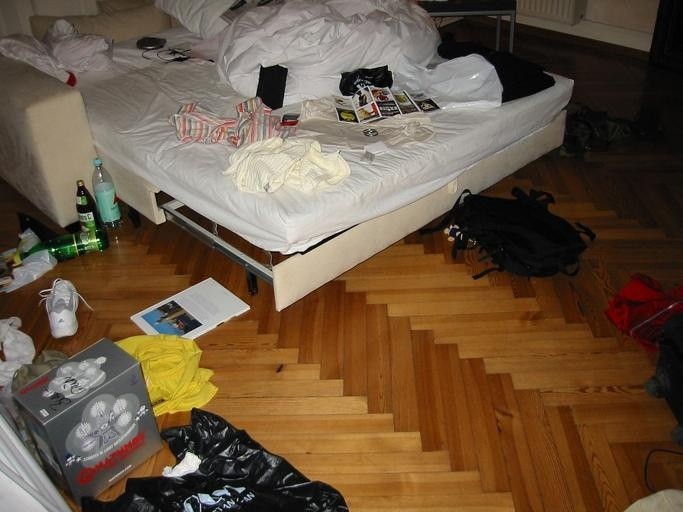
[516,0,587,27]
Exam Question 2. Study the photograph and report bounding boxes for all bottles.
[92,159,124,228]
[74,181,102,233]
[19,227,107,262]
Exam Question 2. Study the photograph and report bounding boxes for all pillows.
[26,2,171,50]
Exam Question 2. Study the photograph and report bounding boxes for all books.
[129,276,251,341]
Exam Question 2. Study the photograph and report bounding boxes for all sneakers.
[45,280,79,339]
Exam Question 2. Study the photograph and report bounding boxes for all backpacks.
[449,186,585,279]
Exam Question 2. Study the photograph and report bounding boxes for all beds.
[2,1,577,313]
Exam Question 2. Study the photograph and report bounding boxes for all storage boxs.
[10,337,165,506]
